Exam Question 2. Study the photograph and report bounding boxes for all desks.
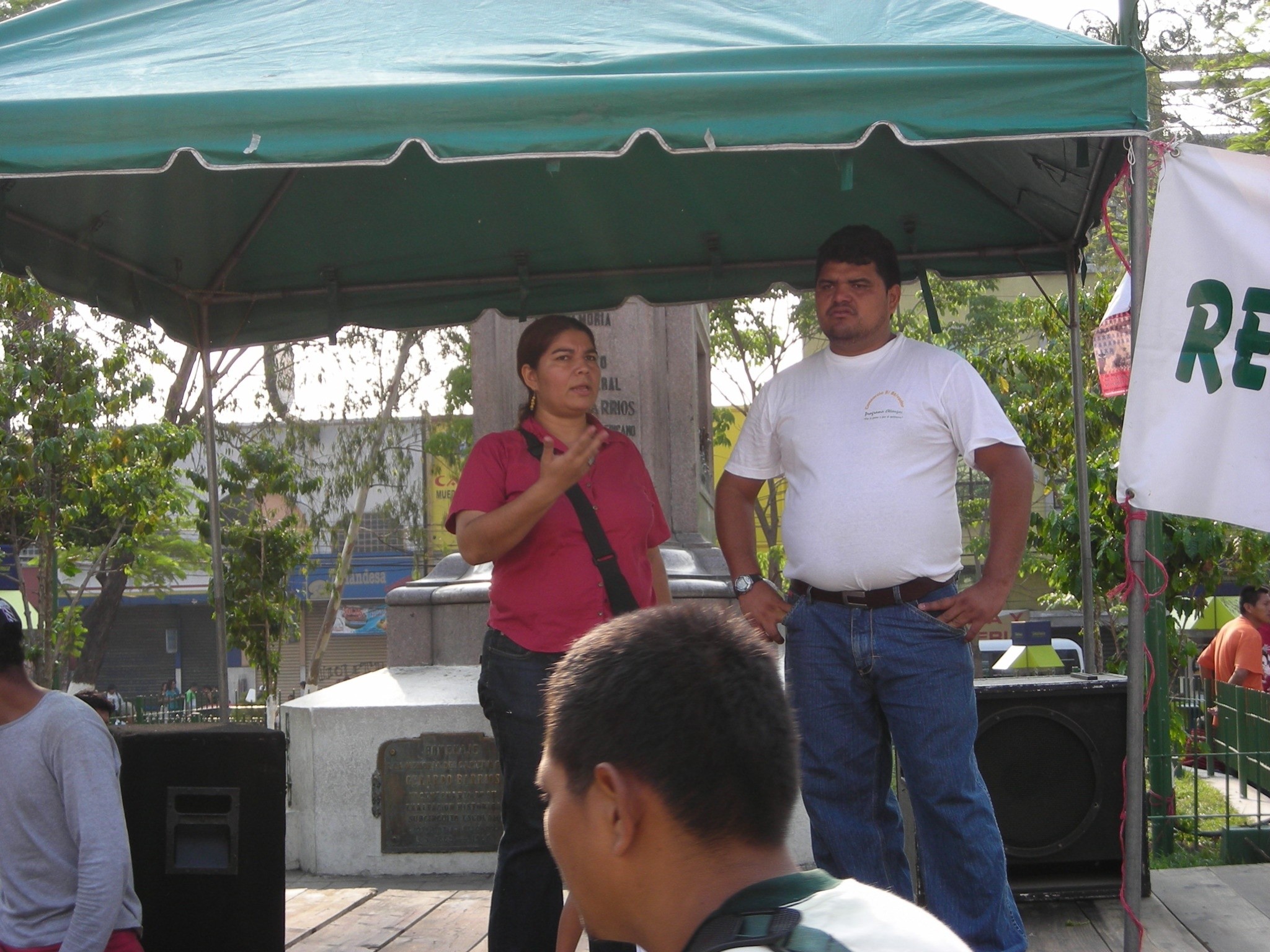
[895,673,1150,902]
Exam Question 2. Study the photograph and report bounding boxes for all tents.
[0,0,1149,916]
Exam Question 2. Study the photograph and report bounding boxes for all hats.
[0,598,22,640]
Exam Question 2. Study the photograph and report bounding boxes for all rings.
[588,457,593,465]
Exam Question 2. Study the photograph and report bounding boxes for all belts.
[789,571,958,608]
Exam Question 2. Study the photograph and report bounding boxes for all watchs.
[733,574,764,599]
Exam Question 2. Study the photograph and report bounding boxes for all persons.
[118,697,135,716]
[161,679,179,716]
[300,681,305,689]
[105,684,124,716]
[444,314,669,952]
[714,224,1034,952]
[1196,585,1270,727]
[555,891,583,952]
[0,599,145,952]
[186,683,197,709]
[288,695,294,700]
[73,691,116,726]
[204,684,213,700]
[537,602,974,952]
[257,685,266,699]
